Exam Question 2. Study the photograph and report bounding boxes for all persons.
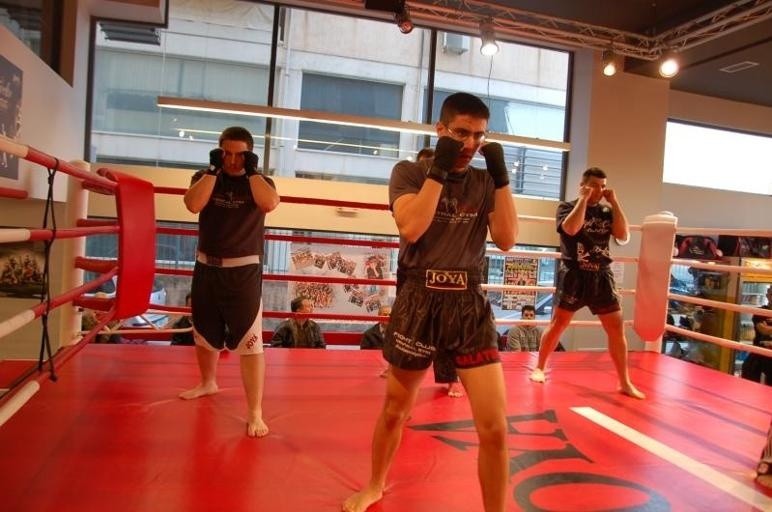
[506,305,541,351]
[742,286,772,386]
[270,296,326,348]
[171,293,194,345]
[529,167,645,399]
[178,127,280,438]
[82,292,122,344]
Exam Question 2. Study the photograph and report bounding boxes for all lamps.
[602,51,617,78]
[391,8,499,58]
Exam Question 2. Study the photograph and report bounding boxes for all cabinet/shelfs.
[697,258,772,375]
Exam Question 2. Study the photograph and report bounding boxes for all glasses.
[445,125,488,143]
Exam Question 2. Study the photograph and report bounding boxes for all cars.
[489,281,554,352]
[665,288,697,354]
[83,256,169,329]
[106,243,186,261]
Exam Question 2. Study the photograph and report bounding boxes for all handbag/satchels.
[676,235,769,259]
